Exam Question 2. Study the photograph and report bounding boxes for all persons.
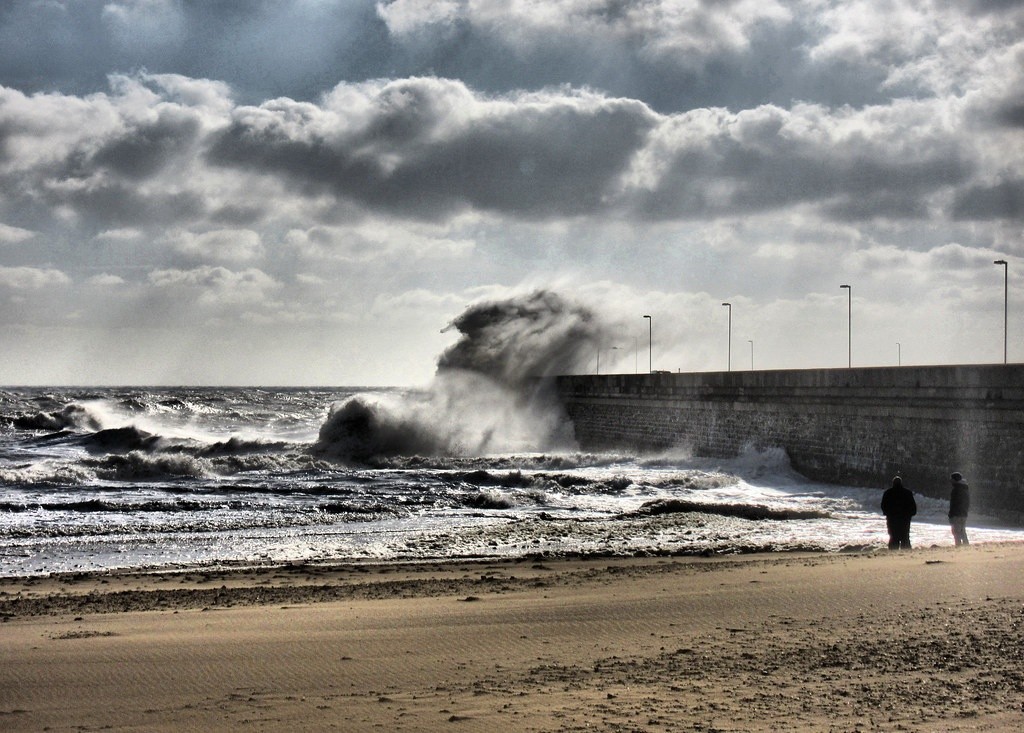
[880,476,917,550]
[948,472,970,548]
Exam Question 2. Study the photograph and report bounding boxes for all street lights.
[840,283,852,367]
[722,302,732,372]
[642,315,651,373]
[994,259,1007,364]
